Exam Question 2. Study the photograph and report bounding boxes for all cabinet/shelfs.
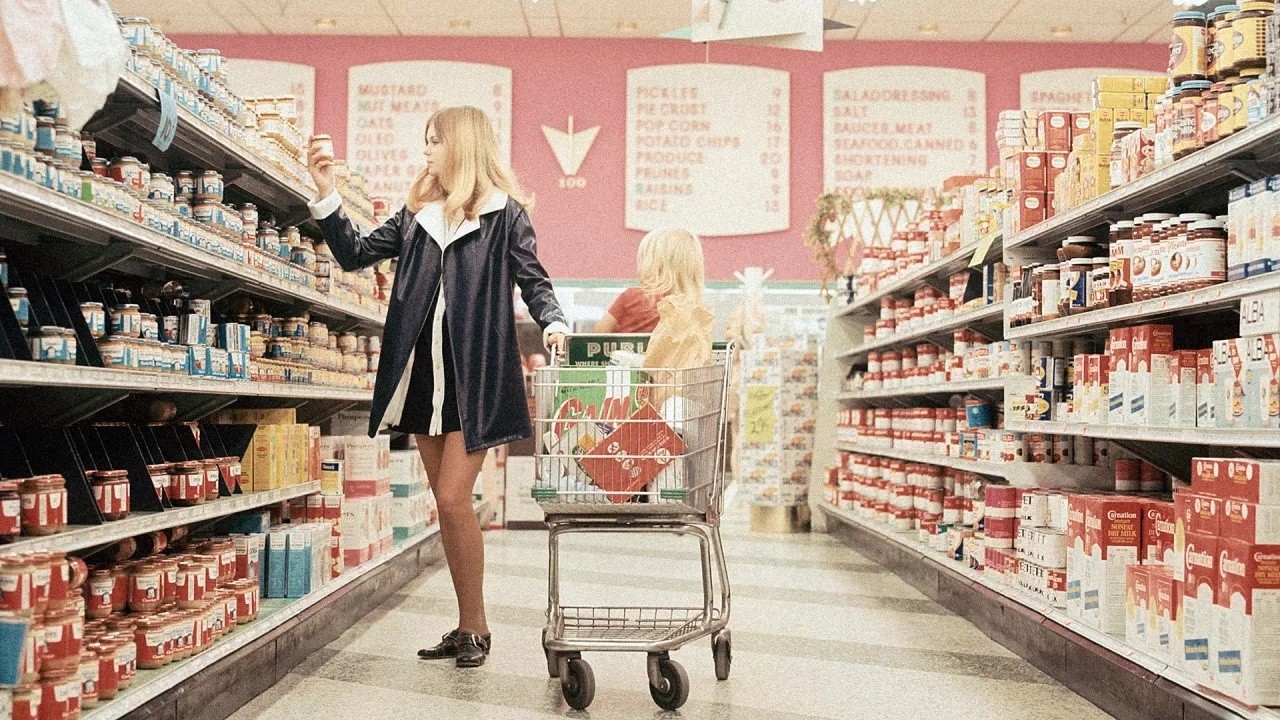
[0,1,502,720]
[807,110,1279,720]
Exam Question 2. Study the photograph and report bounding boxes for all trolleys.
[532,333,736,711]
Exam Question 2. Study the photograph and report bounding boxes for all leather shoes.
[417,628,491,658]
[455,631,488,667]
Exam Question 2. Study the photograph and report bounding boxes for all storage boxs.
[1067,456,1280,706]
[1003,75,1170,231]
[217,408,393,596]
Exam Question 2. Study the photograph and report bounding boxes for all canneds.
[833,0,1280,609]
[0,0,392,720]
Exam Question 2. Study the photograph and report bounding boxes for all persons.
[591,226,705,333]
[307,104,573,667]
